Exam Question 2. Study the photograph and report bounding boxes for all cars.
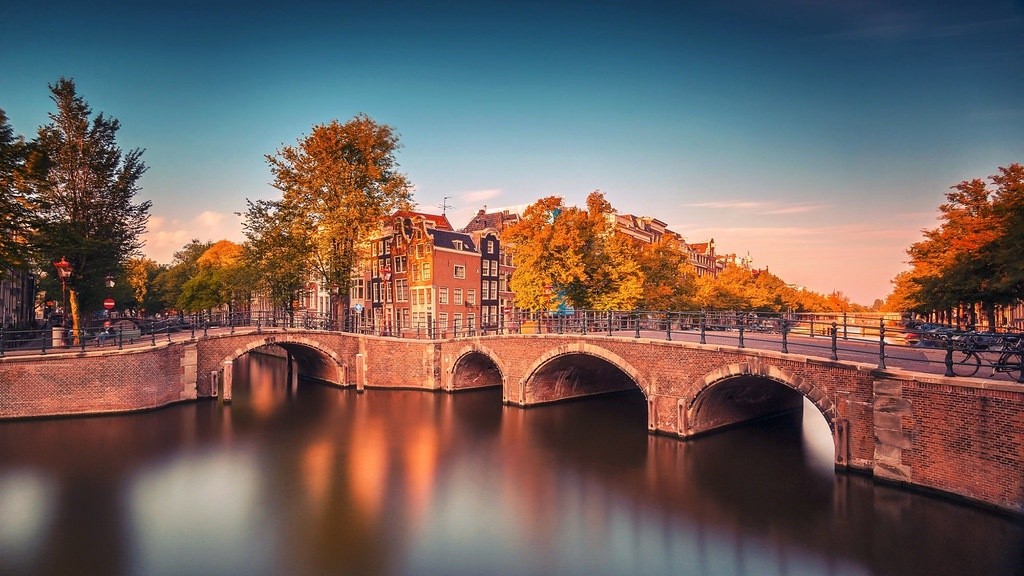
[693,323,769,331]
[901,319,1017,348]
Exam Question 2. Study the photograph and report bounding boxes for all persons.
[92,320,115,348]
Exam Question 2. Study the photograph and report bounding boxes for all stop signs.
[103,299,115,309]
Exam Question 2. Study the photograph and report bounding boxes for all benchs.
[105,329,141,345]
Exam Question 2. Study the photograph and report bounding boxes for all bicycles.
[945,332,1024,381]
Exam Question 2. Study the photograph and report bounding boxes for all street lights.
[54,257,69,327]
[104,272,115,328]
